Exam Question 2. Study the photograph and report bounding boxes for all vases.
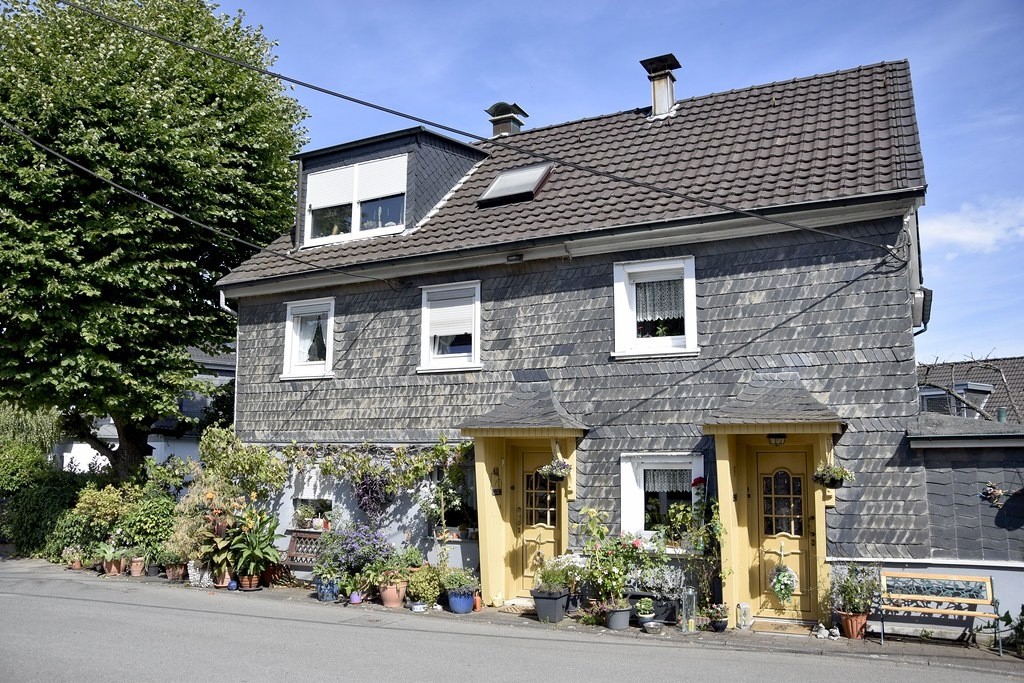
[529,587,569,623]
[606,589,728,635]
[566,596,580,612]
[822,474,843,488]
[71,561,81,570]
[550,474,564,481]
[239,575,263,590]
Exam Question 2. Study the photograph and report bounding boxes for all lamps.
[766,433,787,447]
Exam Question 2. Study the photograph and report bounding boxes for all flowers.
[530,550,572,593]
[574,476,735,633]
[812,459,856,486]
[768,565,799,606]
[60,544,87,566]
[227,491,280,575]
[559,554,588,596]
[536,459,572,477]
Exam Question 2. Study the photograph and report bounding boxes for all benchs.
[879,570,1003,658]
[263,532,323,588]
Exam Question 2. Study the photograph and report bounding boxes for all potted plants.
[311,504,482,614]
[294,504,316,528]
[92,477,233,589]
[836,562,886,640]
[997,603,1024,658]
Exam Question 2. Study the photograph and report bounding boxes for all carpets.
[749,620,814,636]
[498,605,538,615]
